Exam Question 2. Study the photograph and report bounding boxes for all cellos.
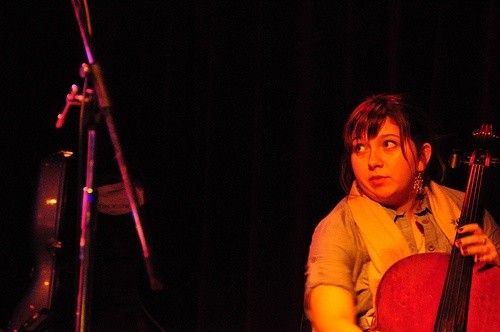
[370,123,500,332]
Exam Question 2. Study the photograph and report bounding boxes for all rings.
[481,236,488,242]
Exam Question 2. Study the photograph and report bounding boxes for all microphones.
[55,84,79,128]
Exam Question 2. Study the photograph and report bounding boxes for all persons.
[302,94,500,332]
